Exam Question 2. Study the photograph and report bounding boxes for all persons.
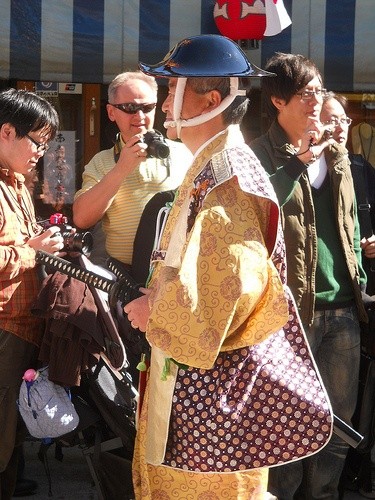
[319,91,375,500]
[132,34,333,499]
[0,87,75,493]
[73,72,196,393]
[247,51,367,500]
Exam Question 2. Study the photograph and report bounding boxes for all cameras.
[311,123,334,146]
[40,223,94,256]
[133,131,170,158]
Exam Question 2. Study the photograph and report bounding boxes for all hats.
[138,33,278,79]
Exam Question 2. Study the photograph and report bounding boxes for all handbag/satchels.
[16,366,80,439]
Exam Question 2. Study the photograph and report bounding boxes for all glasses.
[293,89,327,100]
[27,133,51,152]
[322,116,353,127]
[108,102,157,114]
